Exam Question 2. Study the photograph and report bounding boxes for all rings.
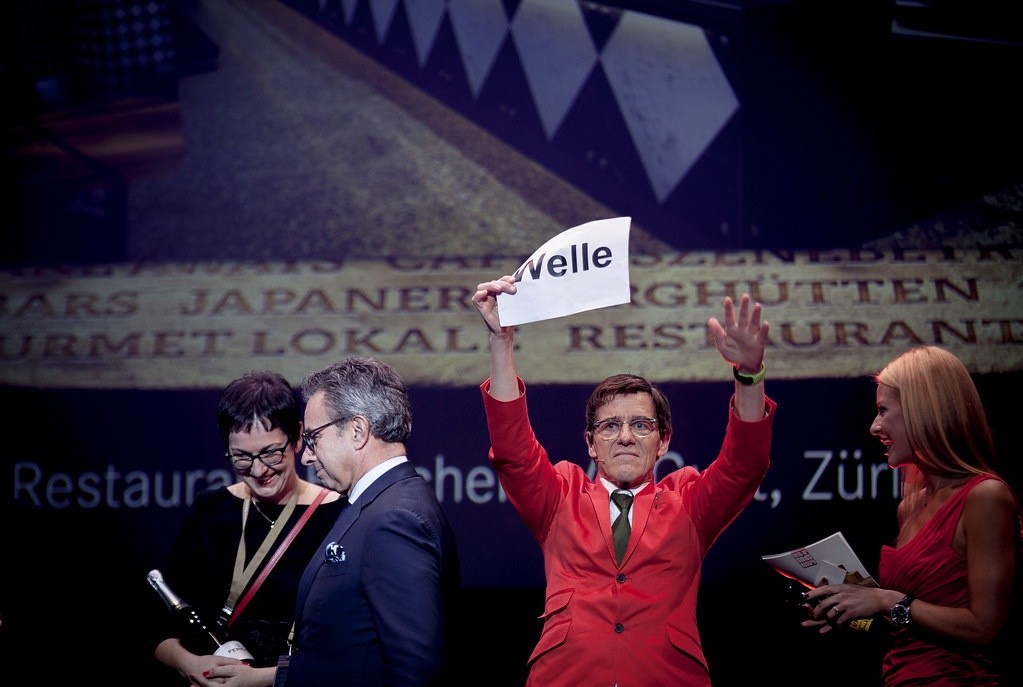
[833,607,840,617]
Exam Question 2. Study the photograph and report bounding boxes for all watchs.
[728,363,768,386]
[886,594,917,627]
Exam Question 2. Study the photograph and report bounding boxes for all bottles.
[147,567,260,669]
[789,584,880,635]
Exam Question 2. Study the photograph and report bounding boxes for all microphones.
[595,459,605,464]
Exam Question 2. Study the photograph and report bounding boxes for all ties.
[611,490,634,567]
[334,499,351,524]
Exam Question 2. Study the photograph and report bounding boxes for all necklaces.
[916,482,953,508]
[251,491,296,529]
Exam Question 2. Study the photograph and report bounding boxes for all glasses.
[300,417,351,452]
[592,414,657,438]
[223,435,293,470]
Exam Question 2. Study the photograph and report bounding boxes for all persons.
[796,343,1022,687]
[474,271,779,687]
[271,358,467,686]
[149,370,350,687]
